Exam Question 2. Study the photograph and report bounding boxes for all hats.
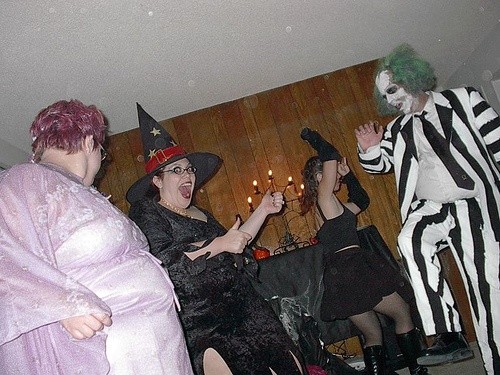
[126,102,224,206]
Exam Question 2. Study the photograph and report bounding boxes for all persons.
[353,43,500,375]
[134,149,307,375]
[0,99,194,375]
[299,129,428,375]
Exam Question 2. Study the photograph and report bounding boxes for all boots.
[397,327,431,375]
[363,345,387,374]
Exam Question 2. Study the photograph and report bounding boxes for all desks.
[257,224,415,349]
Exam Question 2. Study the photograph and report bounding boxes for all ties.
[414,111,475,191]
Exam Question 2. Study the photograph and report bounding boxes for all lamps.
[247,170,310,254]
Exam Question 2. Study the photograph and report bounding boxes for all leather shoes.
[416,331,474,366]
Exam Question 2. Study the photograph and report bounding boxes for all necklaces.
[159,197,197,218]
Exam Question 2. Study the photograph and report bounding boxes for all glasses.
[161,166,196,175]
[96,139,108,161]
